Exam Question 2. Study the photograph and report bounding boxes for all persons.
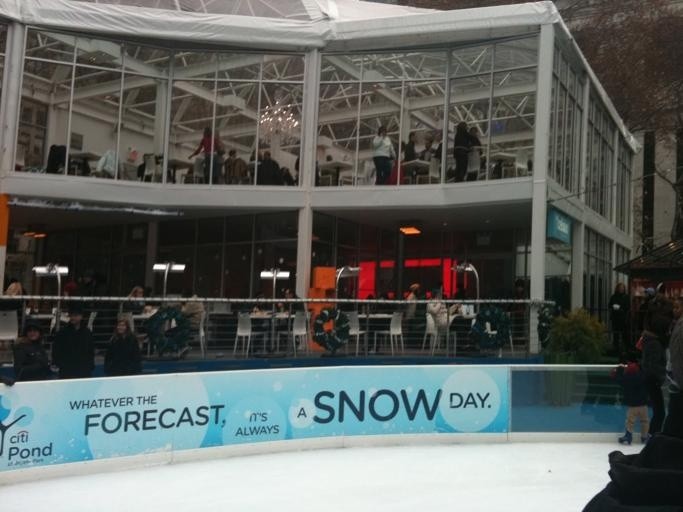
[583,283,683,511]
[510,279,529,348]
[5,281,204,381]
[373,121,502,184]
[359,282,477,353]
[187,126,334,185]
[249,287,304,357]
[138,153,152,183]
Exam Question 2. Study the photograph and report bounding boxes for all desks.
[70,150,519,185]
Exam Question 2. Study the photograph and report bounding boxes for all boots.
[618,432,633,444]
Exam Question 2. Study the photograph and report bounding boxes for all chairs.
[0,310,19,367]
[50,308,207,358]
[234,304,514,356]
